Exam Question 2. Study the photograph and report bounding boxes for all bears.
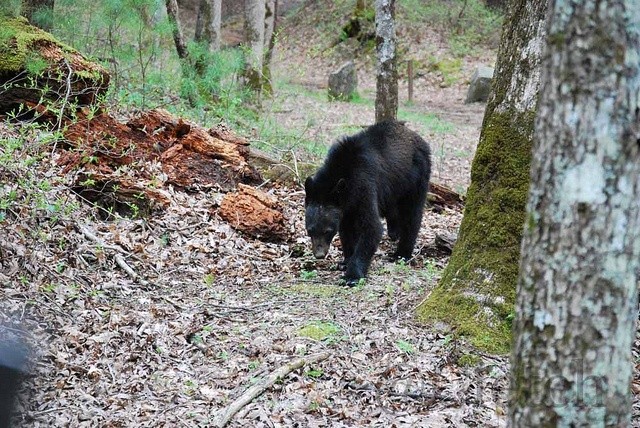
[305,118,433,287]
